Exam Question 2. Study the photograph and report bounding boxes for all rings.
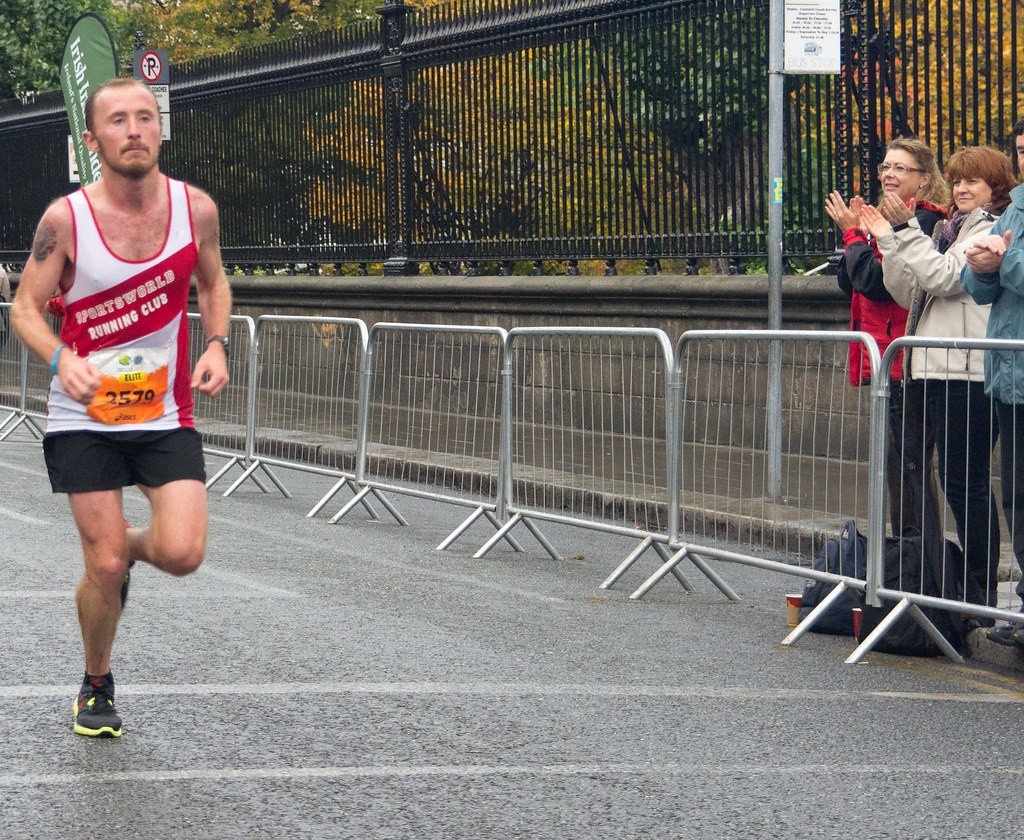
[834,212,837,216]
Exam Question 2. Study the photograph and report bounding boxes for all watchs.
[205,335,229,356]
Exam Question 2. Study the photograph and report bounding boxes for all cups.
[852,607,862,641]
[786,593,802,628]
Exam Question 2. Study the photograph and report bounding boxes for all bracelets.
[52,344,67,374]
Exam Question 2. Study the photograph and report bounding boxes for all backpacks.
[797,520,962,658]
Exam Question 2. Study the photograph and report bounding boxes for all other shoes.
[986,621,1024,646]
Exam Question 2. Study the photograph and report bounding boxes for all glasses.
[877,163,926,175]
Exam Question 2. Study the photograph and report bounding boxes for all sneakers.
[72,676,122,737]
[121,518,134,611]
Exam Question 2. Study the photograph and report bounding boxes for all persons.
[11,78,232,737]
[825,140,1024,646]
